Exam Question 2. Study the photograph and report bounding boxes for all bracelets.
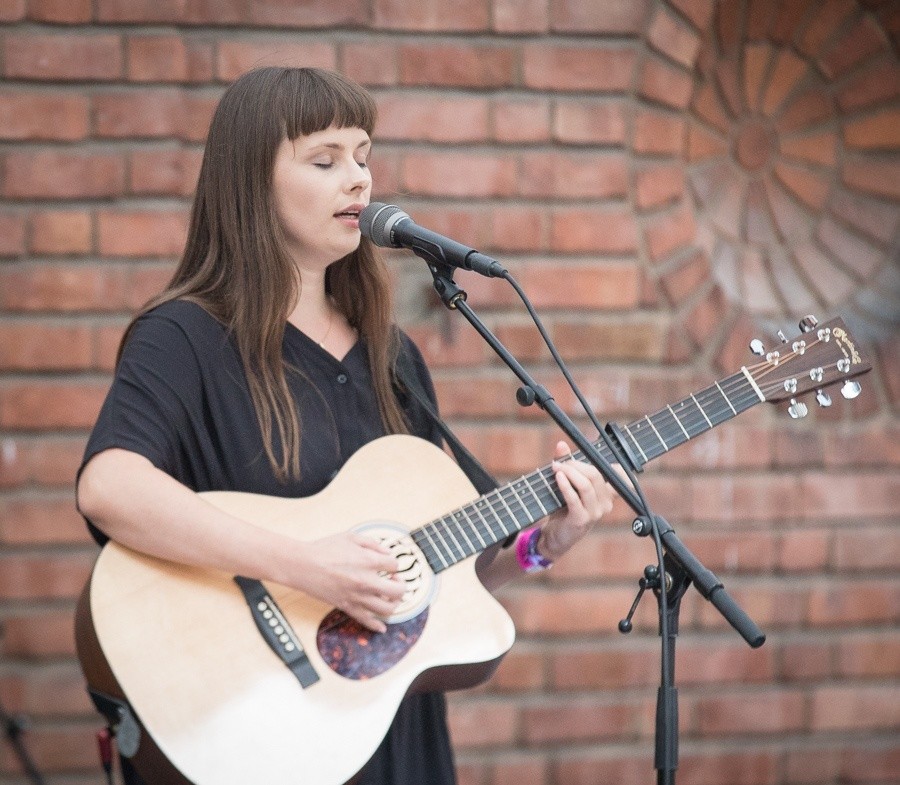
[527,527,554,569]
[516,526,536,572]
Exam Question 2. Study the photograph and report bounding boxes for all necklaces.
[317,298,334,351]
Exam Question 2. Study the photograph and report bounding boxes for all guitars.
[72,316,873,785]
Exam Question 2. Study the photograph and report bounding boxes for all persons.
[74,65,641,785]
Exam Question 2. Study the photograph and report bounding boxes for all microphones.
[356,201,509,280]
